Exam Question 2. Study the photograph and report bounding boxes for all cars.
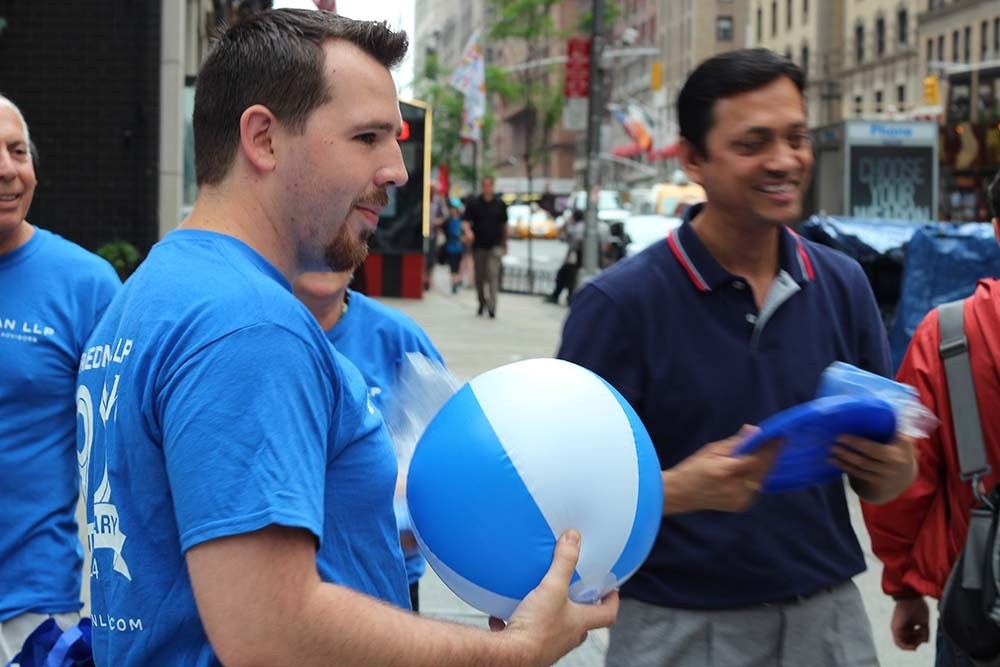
[505,169,706,262]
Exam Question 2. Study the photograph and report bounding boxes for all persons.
[425,179,447,288]
[859,166,1000,667]
[441,197,467,294]
[290,270,446,614]
[544,210,585,305]
[0,97,122,667]
[460,175,509,316]
[555,47,919,667]
[75,8,620,667]
[603,222,632,262]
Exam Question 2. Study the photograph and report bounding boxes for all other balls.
[404,353,668,624]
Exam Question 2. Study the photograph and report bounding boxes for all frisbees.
[730,391,897,497]
